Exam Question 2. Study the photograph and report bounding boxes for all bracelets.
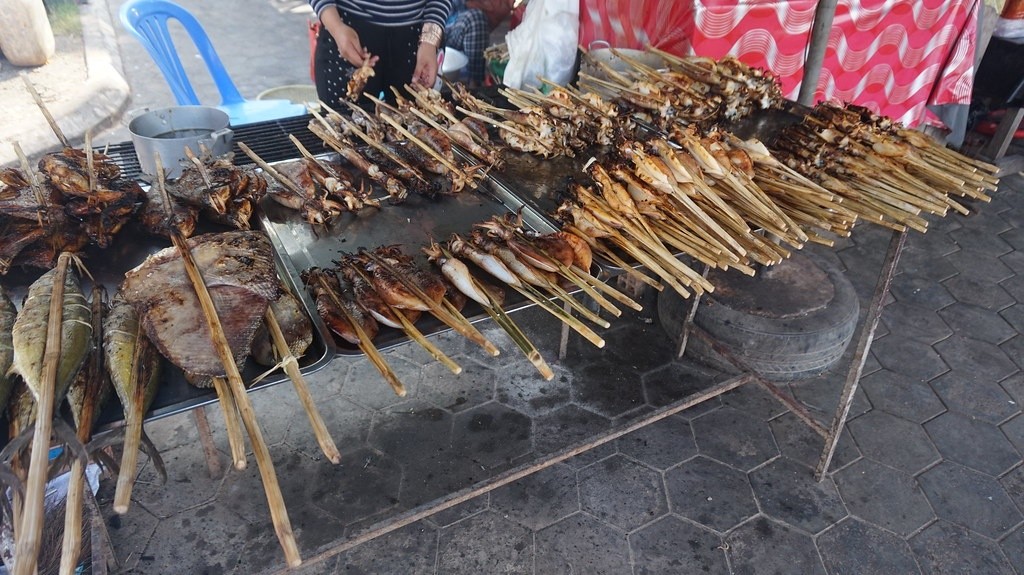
[419,21,441,53]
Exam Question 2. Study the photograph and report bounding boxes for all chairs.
[119,0,308,127]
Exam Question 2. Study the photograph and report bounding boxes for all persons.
[443,0,521,91]
[307,0,453,113]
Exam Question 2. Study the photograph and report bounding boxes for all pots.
[579,40,670,101]
[129,106,233,180]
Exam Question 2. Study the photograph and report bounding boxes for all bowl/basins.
[441,46,469,88]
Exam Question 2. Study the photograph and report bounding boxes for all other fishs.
[0,55,930,517]
